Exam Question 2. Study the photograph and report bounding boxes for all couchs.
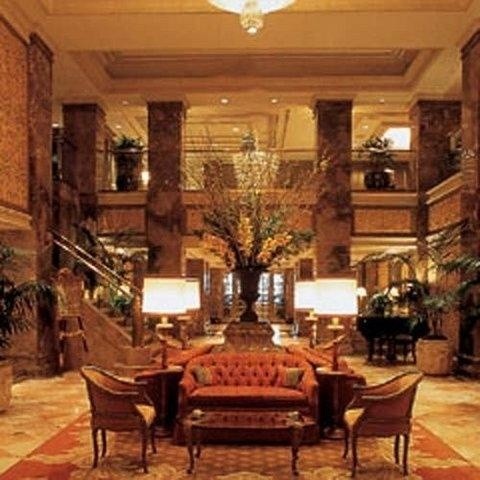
[177,348,323,445]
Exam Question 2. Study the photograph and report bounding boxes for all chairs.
[342,367,425,478]
[75,363,155,478]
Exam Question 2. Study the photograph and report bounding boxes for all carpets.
[1,405,480,480]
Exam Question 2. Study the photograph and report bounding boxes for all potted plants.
[365,216,480,376]
[356,135,393,190]
[1,242,57,413]
[177,109,351,321]
[109,131,147,191]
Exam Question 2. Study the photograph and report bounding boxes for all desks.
[181,410,317,477]
